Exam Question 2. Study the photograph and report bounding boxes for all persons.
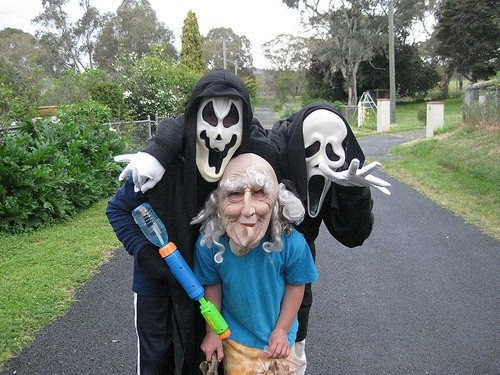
[193,153,318,375]
[106,65,260,374]
[112,100,393,372]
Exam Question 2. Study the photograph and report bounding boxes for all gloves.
[320,157,392,197]
[113,149,165,194]
[132,244,170,286]
